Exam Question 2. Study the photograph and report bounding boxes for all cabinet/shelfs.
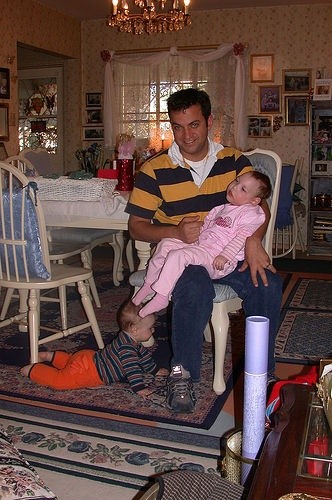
[307,100,332,256]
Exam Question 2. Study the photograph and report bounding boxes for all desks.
[19,191,154,333]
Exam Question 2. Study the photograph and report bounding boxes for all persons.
[123,89,284,414]
[132,171,272,318]
[21,298,169,400]
[261,90,279,111]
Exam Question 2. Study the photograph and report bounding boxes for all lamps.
[107,0,192,35]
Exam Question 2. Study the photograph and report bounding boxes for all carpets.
[0,257,332,500]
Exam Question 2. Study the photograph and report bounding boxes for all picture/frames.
[0,68,10,99]
[0,103,10,141]
[259,84,282,114]
[248,115,273,137]
[312,161,332,175]
[284,95,309,126]
[282,68,313,93]
[83,127,104,140]
[82,108,104,126]
[250,53,274,83]
[85,92,103,107]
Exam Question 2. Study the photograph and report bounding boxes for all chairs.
[0,147,105,364]
[271,163,304,259]
[202,147,282,396]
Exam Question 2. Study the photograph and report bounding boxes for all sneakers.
[167,370,197,414]
[267,373,277,388]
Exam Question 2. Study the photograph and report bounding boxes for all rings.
[266,265,270,269]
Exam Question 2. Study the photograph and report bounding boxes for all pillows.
[0,430,57,500]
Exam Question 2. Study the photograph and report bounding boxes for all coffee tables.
[248,384,332,500]
